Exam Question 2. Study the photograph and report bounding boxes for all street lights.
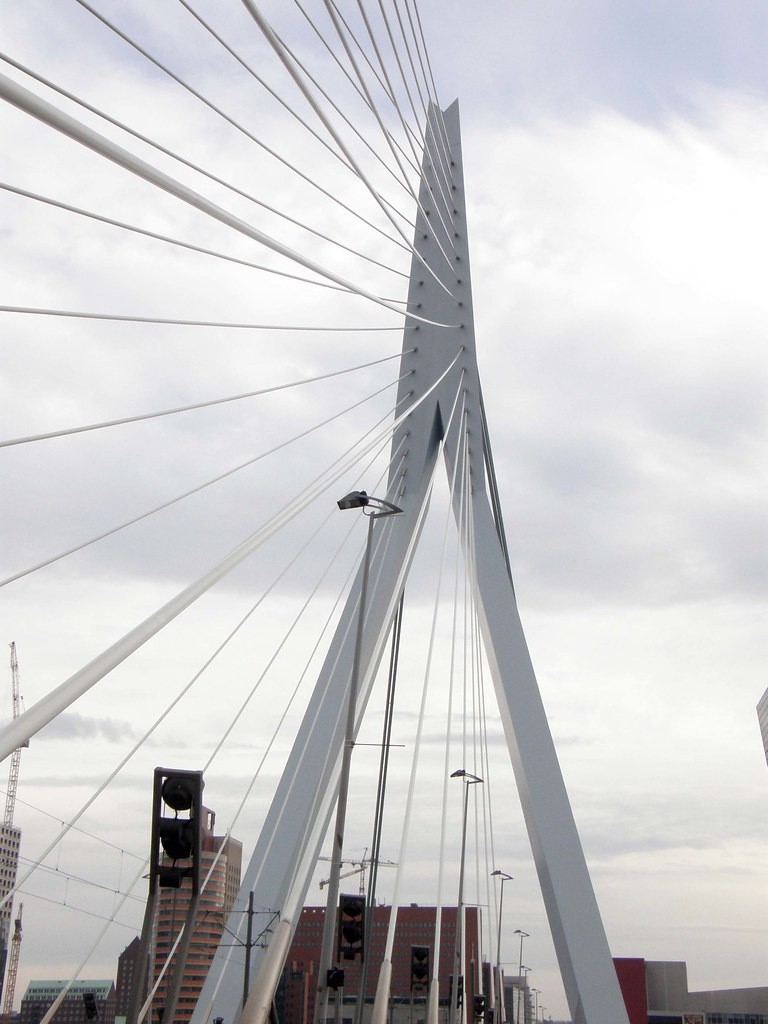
[513,929,530,1024]
[531,988,542,1024]
[448,769,485,1024]
[490,869,514,1024]
[313,490,404,1024]
[521,965,532,1024]
[539,1005,547,1024]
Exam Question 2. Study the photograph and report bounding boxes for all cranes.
[314,846,398,895]
